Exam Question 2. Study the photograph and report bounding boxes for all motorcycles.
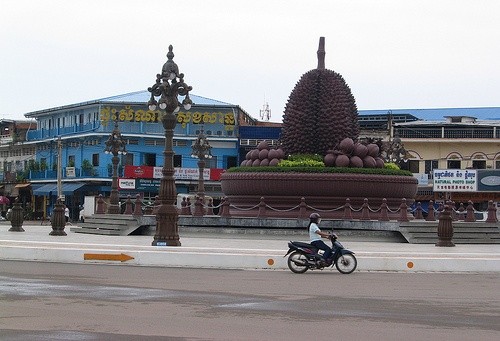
[283,227,357,275]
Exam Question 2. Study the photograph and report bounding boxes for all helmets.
[310,213,321,222]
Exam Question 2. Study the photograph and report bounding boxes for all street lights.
[190,114,213,214]
[384,123,409,168]
[102,111,126,214]
[148,44,193,247]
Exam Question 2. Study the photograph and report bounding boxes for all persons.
[308,213,332,262]
[64,204,84,224]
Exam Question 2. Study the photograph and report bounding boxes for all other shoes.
[320,259,330,266]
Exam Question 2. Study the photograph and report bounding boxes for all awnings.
[33,183,87,195]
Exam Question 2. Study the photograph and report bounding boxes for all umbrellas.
[0,196,10,204]
